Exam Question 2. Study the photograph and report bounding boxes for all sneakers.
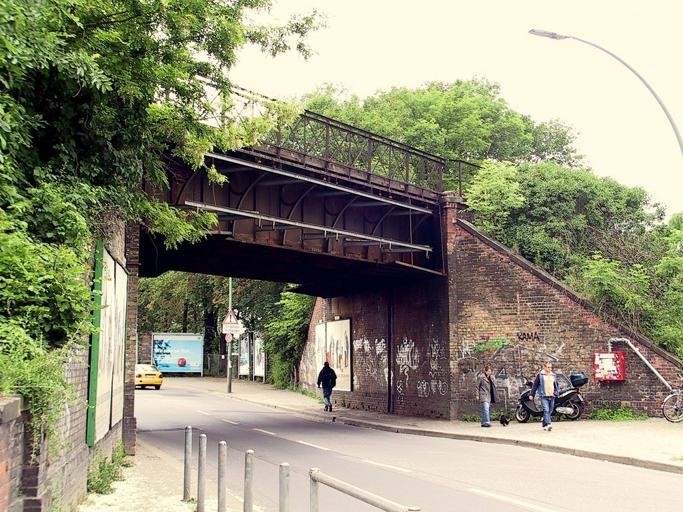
[481,423,490,427]
[324,407,328,411]
[547,425,552,431]
[328,403,332,412]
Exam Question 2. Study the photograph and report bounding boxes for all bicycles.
[660,384,683,424]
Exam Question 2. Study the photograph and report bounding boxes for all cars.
[135,364,164,390]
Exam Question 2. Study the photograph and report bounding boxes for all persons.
[474,365,500,427]
[316,360,337,413]
[527,360,560,432]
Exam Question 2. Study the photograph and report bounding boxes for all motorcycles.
[514,370,589,423]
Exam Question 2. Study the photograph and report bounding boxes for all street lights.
[528,28,683,157]
